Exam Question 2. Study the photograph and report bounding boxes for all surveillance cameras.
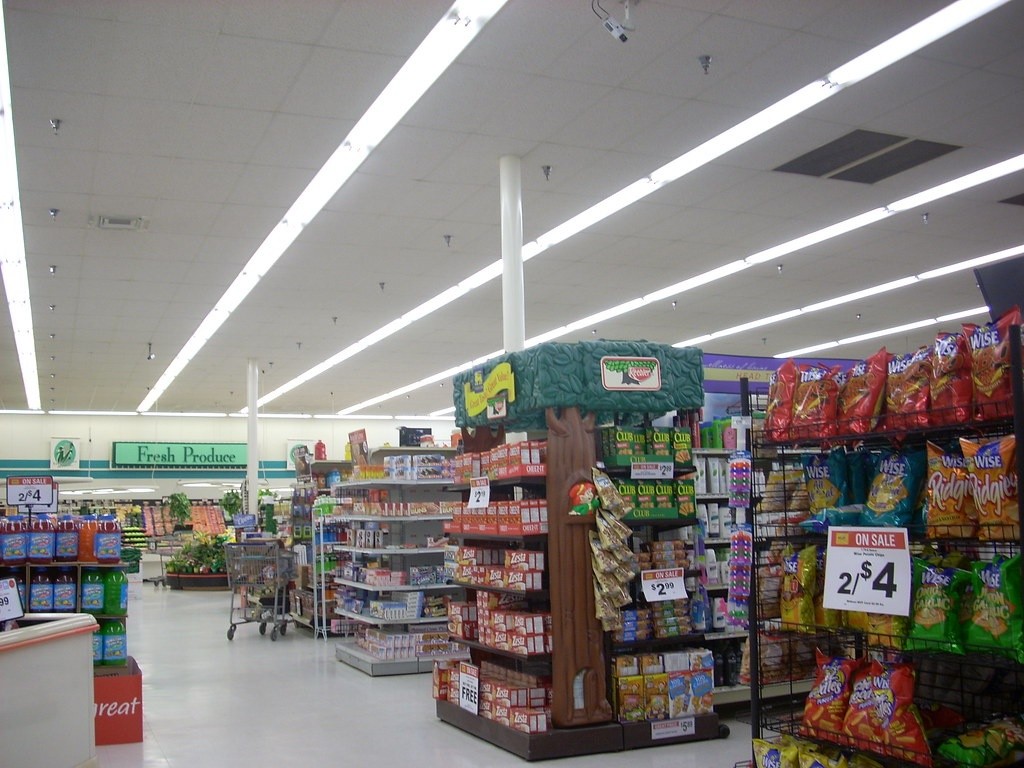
[602,16,629,43]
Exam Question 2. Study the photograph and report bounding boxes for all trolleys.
[221,542,298,642]
[147,545,180,587]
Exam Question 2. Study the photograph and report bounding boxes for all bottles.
[312,467,353,489]
[713,649,743,686]
[92,617,127,666]
[317,573,345,627]
[316,548,351,572]
[344,442,352,460]
[0,513,122,566]
[315,440,327,460]
[315,519,350,545]
[313,493,369,516]
[3,566,128,616]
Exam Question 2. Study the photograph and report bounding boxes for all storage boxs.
[336,454,470,658]
[435,428,694,735]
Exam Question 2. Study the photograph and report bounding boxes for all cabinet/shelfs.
[433,438,886,761]
[289,458,357,641]
[330,477,472,677]
[2,562,131,670]
